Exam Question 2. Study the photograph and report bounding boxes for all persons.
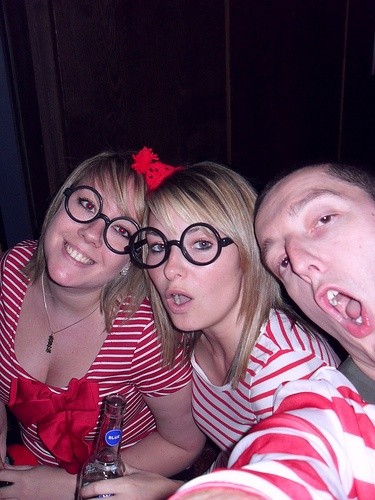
[0,152,206,500]
[80,162,375,500]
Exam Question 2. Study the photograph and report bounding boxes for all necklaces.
[41,265,101,353]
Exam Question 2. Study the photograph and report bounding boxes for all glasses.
[63,185,141,255]
[124,223,235,269]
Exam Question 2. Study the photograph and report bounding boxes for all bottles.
[72,393,129,500]
